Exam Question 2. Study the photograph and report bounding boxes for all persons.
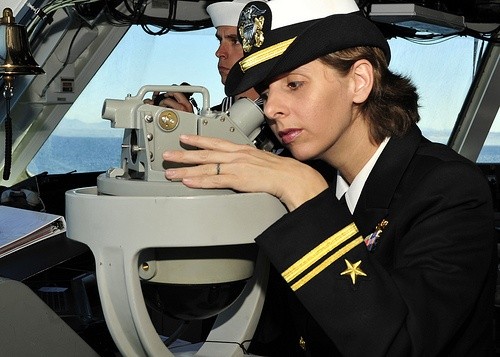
[143,1,291,156]
[163,1,500,357]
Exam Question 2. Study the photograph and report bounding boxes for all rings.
[217,162,220,175]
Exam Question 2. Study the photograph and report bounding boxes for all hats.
[206,0,253,27]
[224,0,391,97]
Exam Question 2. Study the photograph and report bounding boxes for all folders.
[0,205,67,259]
